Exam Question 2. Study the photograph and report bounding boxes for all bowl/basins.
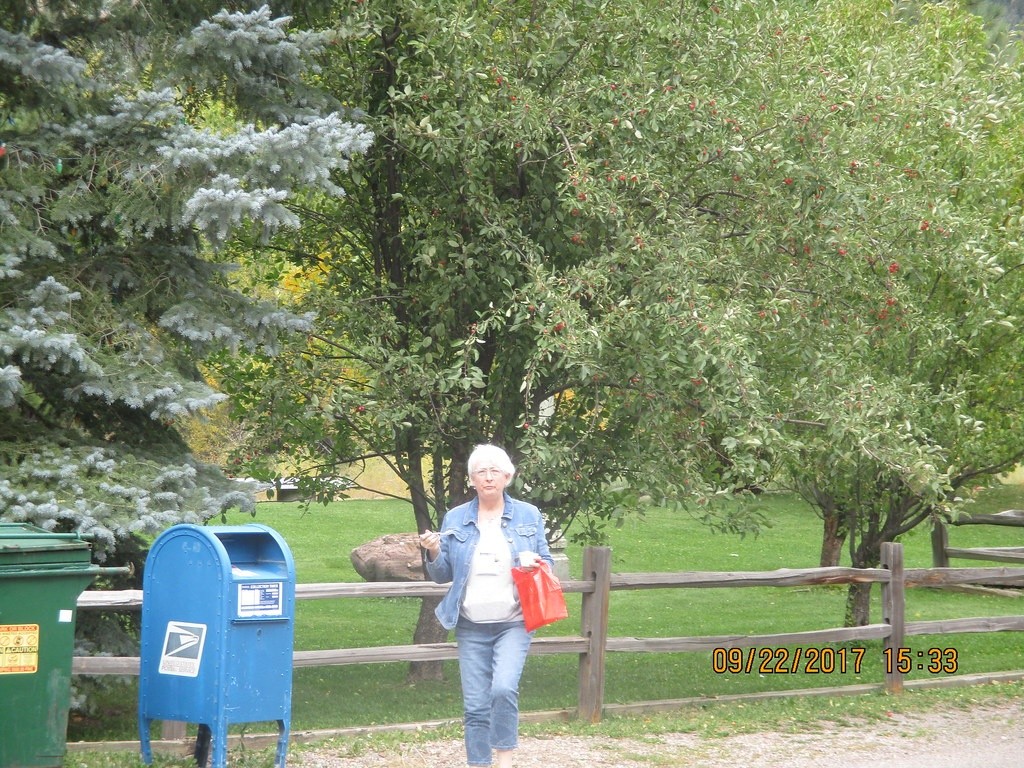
[518,552,539,567]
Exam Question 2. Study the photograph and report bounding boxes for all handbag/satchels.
[510,561,568,633]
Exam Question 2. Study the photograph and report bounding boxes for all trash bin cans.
[138,523,297,768]
[0,523,131,768]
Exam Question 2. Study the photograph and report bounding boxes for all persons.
[419,444,555,767]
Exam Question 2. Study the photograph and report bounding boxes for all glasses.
[471,467,504,476]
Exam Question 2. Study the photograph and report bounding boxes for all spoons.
[419,529,454,537]
